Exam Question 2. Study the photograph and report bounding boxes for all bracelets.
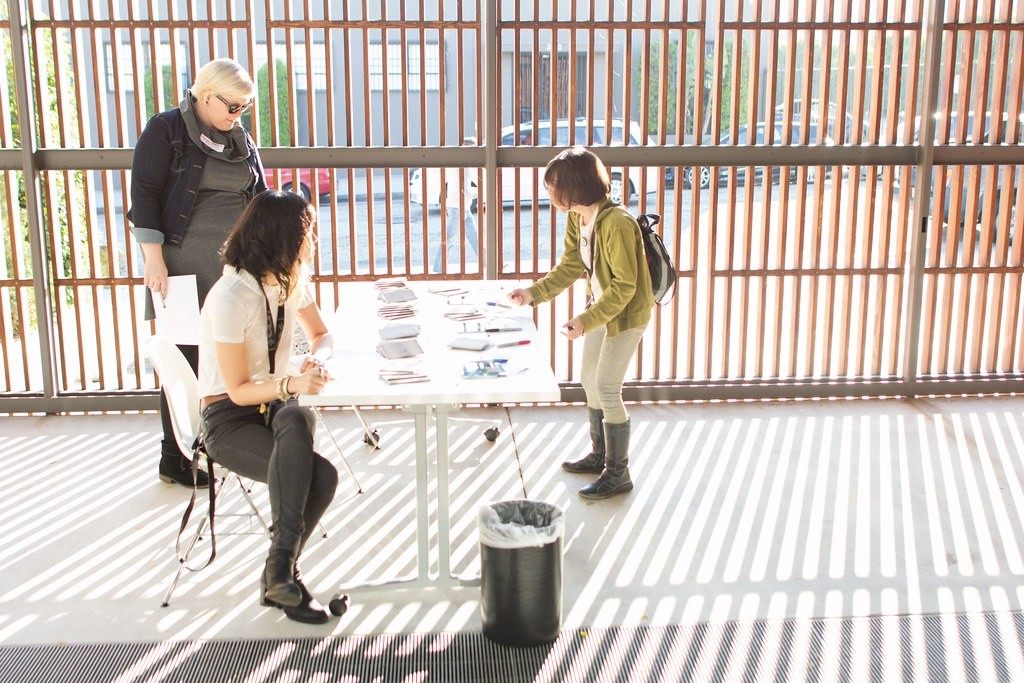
[277,376,297,403]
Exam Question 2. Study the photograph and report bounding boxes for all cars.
[264,166,332,206]
[410,116,658,218]
[764,94,1024,248]
[664,121,835,190]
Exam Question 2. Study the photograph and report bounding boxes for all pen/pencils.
[486,327,522,332]
[510,293,516,300]
[487,301,512,311]
[496,340,531,348]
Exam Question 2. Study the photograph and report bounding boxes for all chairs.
[145,334,272,609]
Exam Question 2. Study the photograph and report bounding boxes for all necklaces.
[581,225,587,246]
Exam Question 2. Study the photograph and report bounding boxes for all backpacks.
[610,200,680,307]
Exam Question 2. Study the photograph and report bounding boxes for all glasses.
[217,96,250,115]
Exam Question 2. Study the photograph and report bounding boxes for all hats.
[523,135,532,143]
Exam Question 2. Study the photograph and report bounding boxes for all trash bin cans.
[480,500,565,646]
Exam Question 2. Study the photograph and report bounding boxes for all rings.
[319,368,323,376]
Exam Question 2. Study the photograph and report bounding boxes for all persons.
[505,144,653,501]
[197,189,339,624]
[124,58,267,488]
[431,137,510,275]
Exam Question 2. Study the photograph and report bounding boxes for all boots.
[157,441,218,490]
[562,406,606,475]
[258,547,328,624]
[576,416,634,499]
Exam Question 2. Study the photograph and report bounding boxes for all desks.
[297,279,562,615]
